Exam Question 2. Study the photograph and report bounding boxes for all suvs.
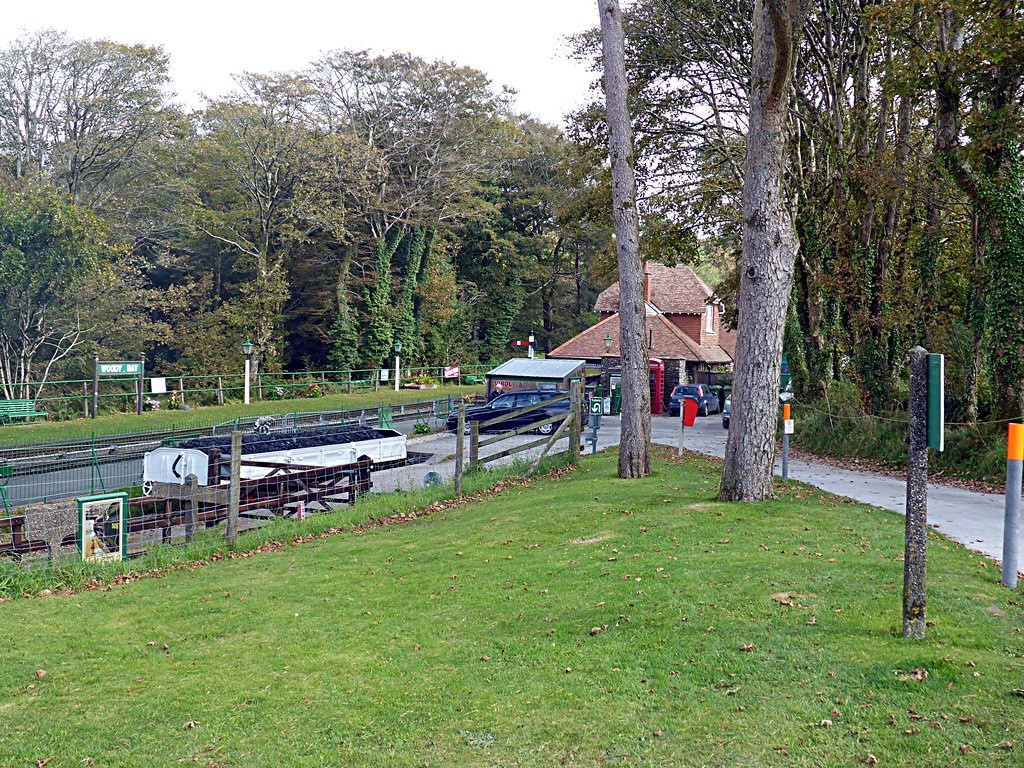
[446,388,589,435]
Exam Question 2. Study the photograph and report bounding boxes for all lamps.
[604,334,613,353]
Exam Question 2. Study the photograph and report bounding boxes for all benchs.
[0,401,49,426]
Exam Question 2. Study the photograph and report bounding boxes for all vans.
[780,356,791,390]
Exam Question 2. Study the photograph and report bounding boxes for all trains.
[93,502,119,550]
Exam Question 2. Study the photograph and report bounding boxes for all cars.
[669,384,720,417]
[781,379,796,405]
[722,393,732,428]
